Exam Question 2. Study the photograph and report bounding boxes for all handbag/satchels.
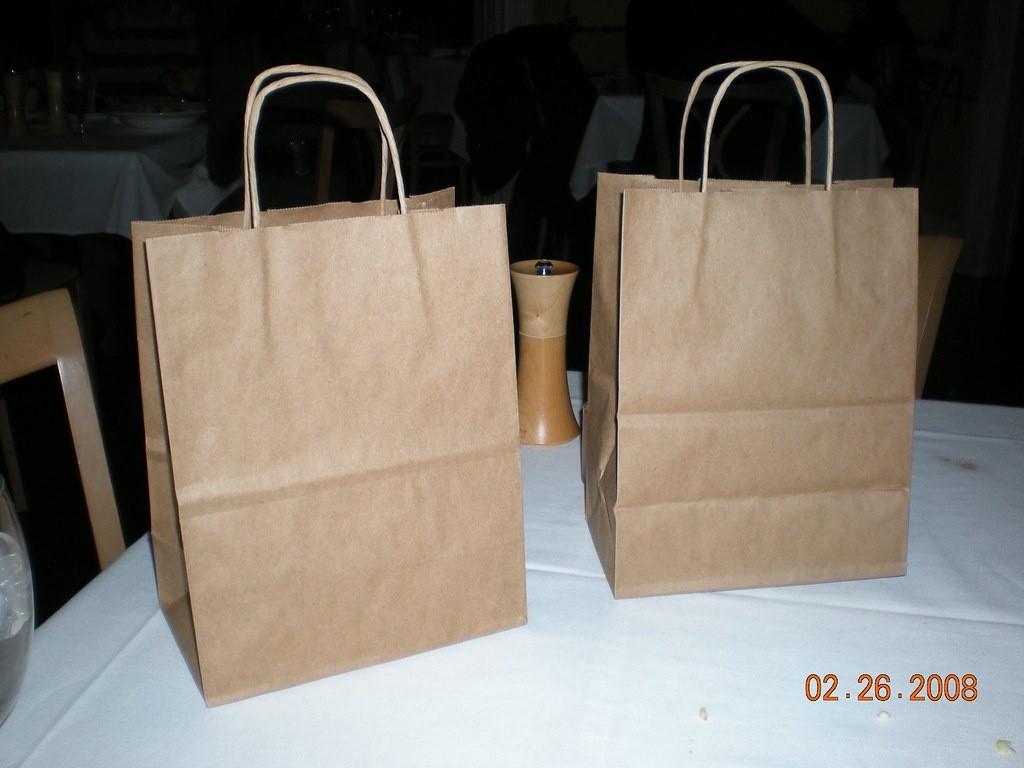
[130,65,529,709]
[581,59,920,600]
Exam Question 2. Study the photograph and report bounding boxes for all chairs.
[639,63,803,180]
[906,37,964,188]
[449,22,600,264]
[78,0,205,115]
[0,287,127,572]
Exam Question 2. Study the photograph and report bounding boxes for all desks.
[351,45,547,203]
[0,120,245,240]
[0,371,1024,768]
[385,54,549,211]
